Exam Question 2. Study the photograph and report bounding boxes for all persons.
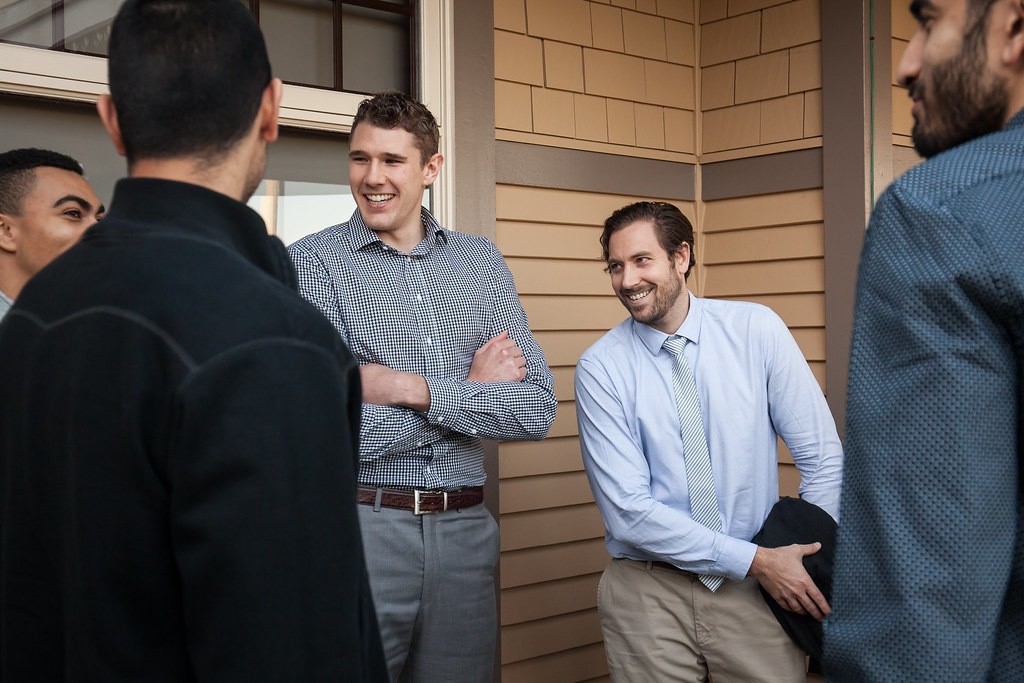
[0,0,391,682]
[574,202,845,683]
[821,0,1024,683]
[283,93,556,683]
[1,147,105,319]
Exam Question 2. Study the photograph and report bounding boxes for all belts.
[356,485,484,515]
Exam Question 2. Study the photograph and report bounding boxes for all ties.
[662,336,726,594]
